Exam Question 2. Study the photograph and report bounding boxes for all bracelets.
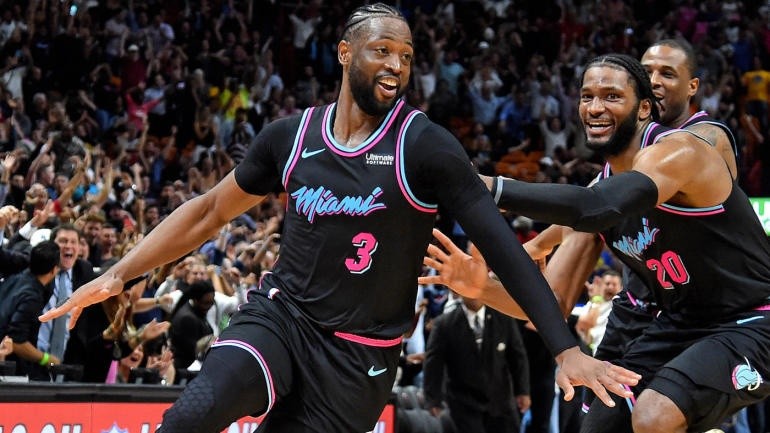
[156,297,160,304]
[39,353,49,365]
[136,335,141,343]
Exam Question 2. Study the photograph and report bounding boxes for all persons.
[520,40,739,420]
[36,4,642,433]
[0,0,770,433]
[418,55,770,433]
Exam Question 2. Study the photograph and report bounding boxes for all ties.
[50,270,67,363]
[472,315,483,352]
